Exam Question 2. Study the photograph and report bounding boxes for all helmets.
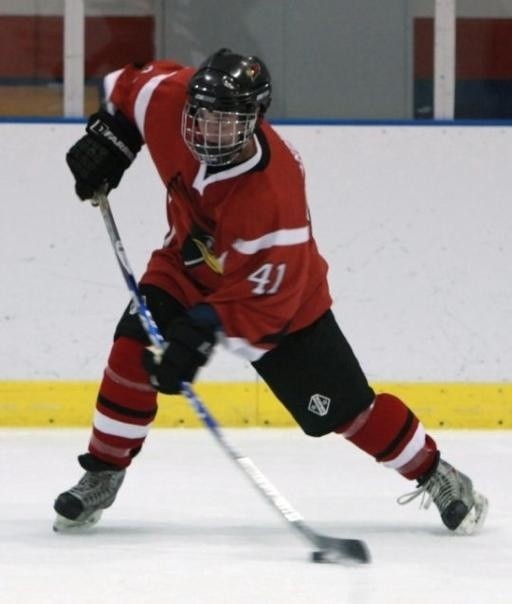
[185,45,276,138]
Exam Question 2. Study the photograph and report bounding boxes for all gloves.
[140,304,221,397]
[58,107,144,204]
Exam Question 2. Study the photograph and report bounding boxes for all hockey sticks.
[95,192,370,564]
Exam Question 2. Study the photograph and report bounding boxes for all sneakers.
[413,448,476,532]
[51,451,127,521]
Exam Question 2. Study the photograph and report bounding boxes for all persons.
[51,47,477,534]
